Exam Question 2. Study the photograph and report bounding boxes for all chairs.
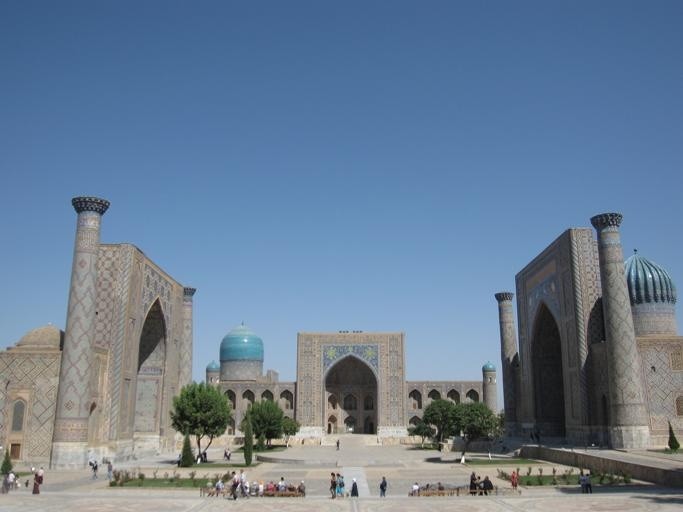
[412,485,496,497]
[200,488,304,498]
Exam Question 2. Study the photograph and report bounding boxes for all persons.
[203,451,207,463]
[510,471,519,491]
[177,454,181,467]
[580,472,592,494]
[329,473,358,498]
[223,449,231,461]
[216,469,305,500]
[3,467,43,494]
[300,439,305,446]
[470,472,493,496]
[90,461,112,480]
[410,482,444,496]
[336,439,340,449]
[380,477,387,497]
